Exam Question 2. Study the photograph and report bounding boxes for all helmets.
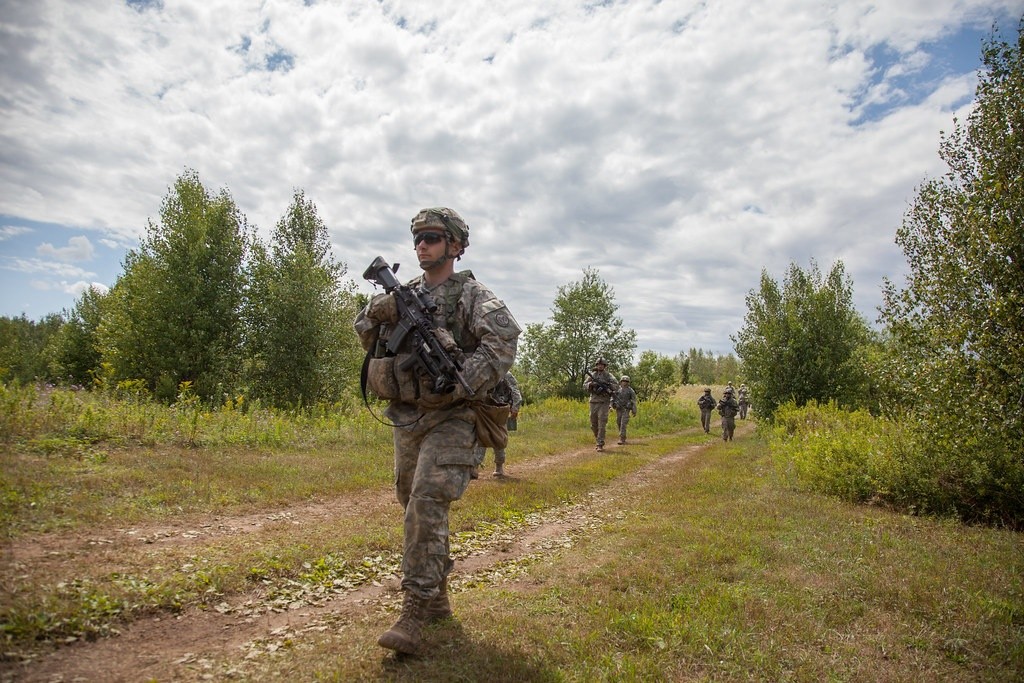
[595,357,607,367]
[620,375,630,383]
[724,389,732,394]
[411,207,469,248]
[704,388,711,392]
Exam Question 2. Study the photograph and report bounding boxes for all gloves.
[381,291,402,325]
[417,377,465,414]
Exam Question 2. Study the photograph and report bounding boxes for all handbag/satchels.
[474,393,510,448]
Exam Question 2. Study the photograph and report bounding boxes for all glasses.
[414,232,446,245]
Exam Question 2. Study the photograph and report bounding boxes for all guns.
[581,369,624,403]
[362,256,477,399]
[700,396,715,410]
[718,399,740,412]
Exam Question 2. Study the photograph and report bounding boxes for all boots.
[470,466,478,479]
[377,588,431,654]
[424,576,451,617]
[493,463,503,475]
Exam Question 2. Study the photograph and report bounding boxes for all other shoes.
[723,439,728,443]
[729,436,732,441]
[704,430,709,435]
[617,436,625,444]
[595,444,603,452]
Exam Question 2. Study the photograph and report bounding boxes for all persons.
[739,390,752,421]
[356,206,520,660]
[739,383,748,397]
[612,375,639,447]
[718,390,740,443]
[467,370,523,482]
[725,381,737,396]
[697,388,718,435]
[584,356,620,452]
